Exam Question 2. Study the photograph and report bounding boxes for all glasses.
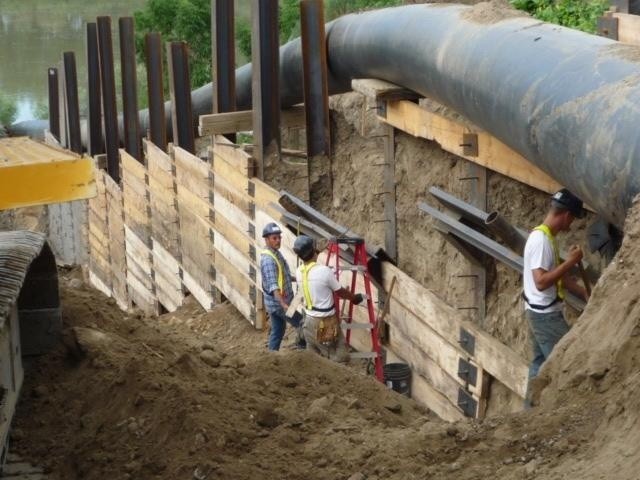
[271,236,281,240]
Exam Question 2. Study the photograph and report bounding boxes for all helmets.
[261,223,281,237]
[292,236,315,259]
[549,190,586,219]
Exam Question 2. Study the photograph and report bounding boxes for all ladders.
[325,237,385,383]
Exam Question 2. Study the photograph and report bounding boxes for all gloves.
[353,294,362,304]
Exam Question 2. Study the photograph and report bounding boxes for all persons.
[290,235,365,367]
[257,222,303,351]
[519,185,585,413]
